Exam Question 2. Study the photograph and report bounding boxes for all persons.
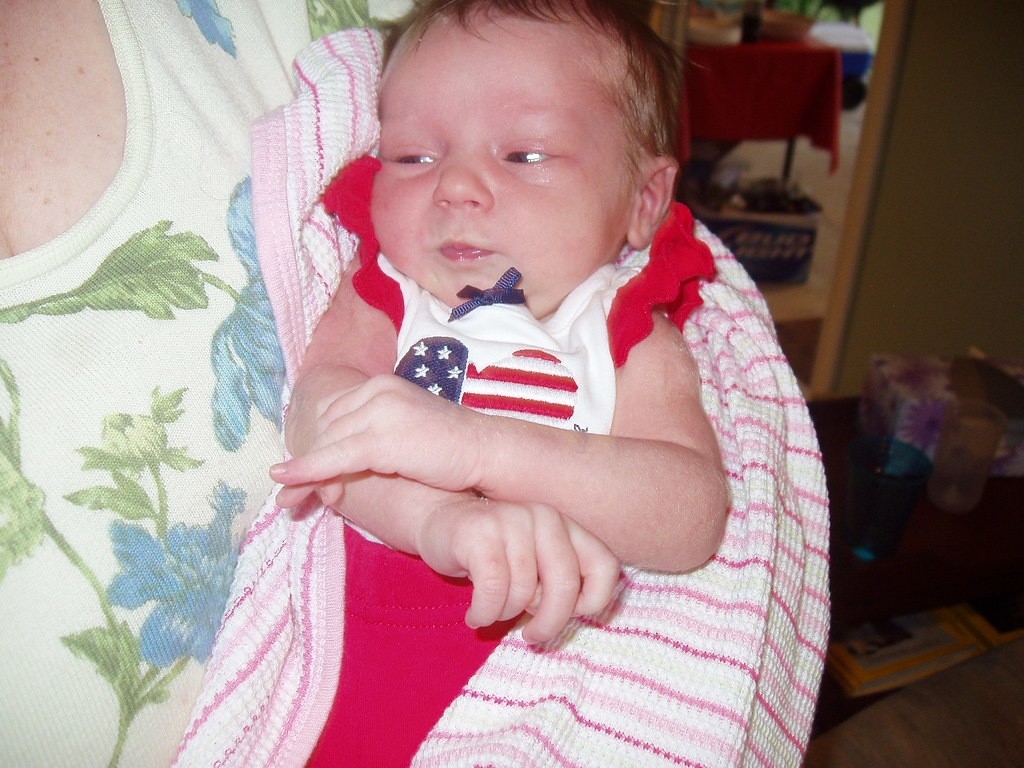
[167,0,830,768]
[0,1,414,768]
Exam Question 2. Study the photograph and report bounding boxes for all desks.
[802,392,1024,768]
[675,36,844,188]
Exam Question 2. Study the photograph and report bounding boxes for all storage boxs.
[689,196,824,284]
